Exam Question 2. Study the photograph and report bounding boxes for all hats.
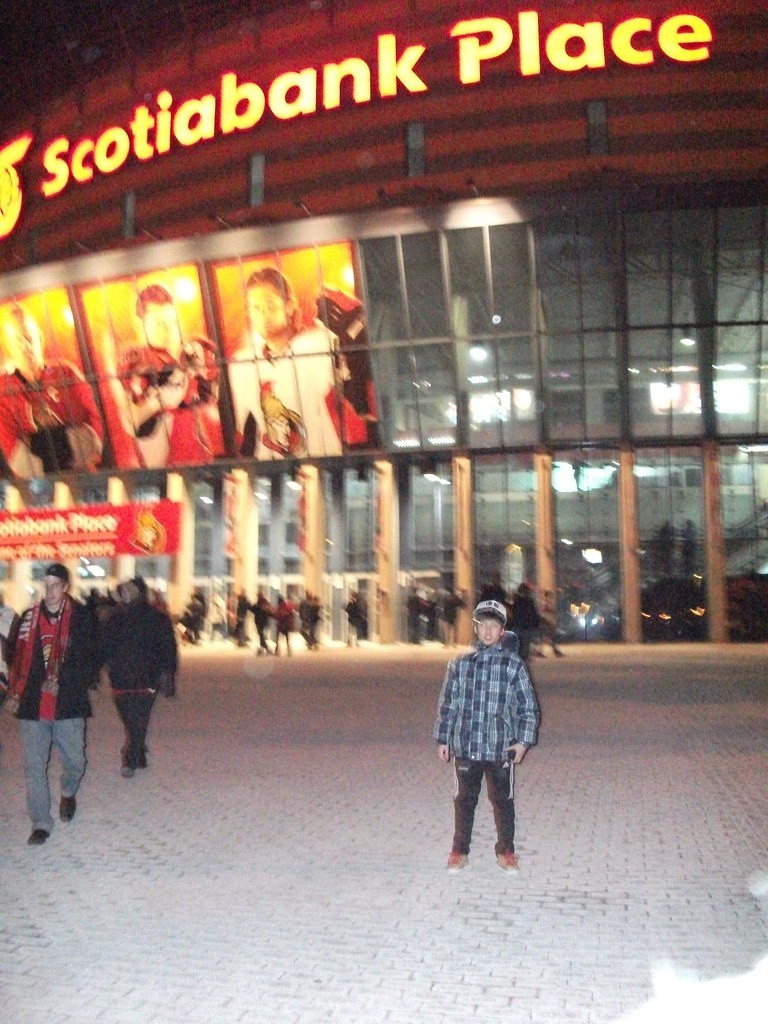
[473,599,508,623]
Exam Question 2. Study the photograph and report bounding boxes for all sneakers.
[498,851,519,873]
[447,852,468,871]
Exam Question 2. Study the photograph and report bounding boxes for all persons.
[86,574,178,778]
[0,571,563,709]
[0,302,108,476]
[434,601,541,873]
[112,287,225,471]
[226,266,379,461]
[7,563,97,846]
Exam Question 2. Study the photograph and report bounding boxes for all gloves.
[162,674,176,699]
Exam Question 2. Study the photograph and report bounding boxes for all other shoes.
[59,795,75,822]
[28,828,49,845]
[120,765,133,778]
